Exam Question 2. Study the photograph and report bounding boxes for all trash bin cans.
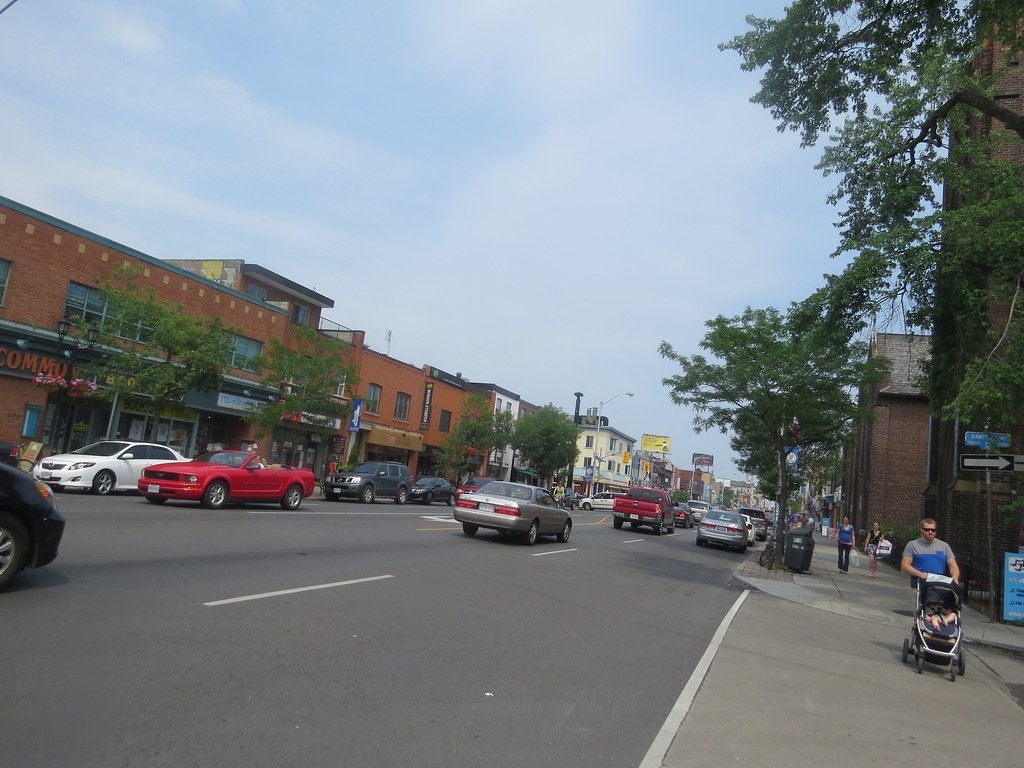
[784,526,815,572]
[827,527,836,538]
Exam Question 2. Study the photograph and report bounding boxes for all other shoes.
[839,569,847,574]
[868,571,876,578]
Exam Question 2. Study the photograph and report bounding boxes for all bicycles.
[759,532,776,569]
[553,495,565,509]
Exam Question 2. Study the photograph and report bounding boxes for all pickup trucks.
[579,492,626,510]
[687,500,710,522]
[613,485,676,535]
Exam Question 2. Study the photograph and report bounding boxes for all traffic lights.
[644,462,650,472]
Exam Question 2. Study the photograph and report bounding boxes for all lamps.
[63,350,73,359]
[244,375,255,395]
[15,316,35,349]
[268,386,281,401]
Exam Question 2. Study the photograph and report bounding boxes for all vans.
[739,508,768,541]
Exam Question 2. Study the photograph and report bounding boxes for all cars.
[138,449,315,510]
[454,474,572,545]
[675,502,695,528]
[765,512,774,525]
[32,441,192,495]
[696,510,756,552]
[408,474,454,505]
[0,442,66,591]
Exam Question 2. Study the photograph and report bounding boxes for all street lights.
[49,313,100,455]
[590,392,634,496]
[690,454,707,499]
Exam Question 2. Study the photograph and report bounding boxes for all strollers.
[902,573,966,681]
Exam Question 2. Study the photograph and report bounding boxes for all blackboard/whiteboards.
[17,441,43,472]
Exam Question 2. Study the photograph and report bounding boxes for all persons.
[252,454,264,469]
[553,483,564,504]
[864,521,883,577]
[796,516,806,527]
[900,518,960,617]
[925,603,956,631]
[835,516,855,574]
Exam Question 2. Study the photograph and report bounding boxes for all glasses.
[921,527,937,532]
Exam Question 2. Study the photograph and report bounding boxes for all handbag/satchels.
[848,549,859,566]
[876,538,892,556]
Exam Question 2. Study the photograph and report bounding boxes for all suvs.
[325,460,413,504]
